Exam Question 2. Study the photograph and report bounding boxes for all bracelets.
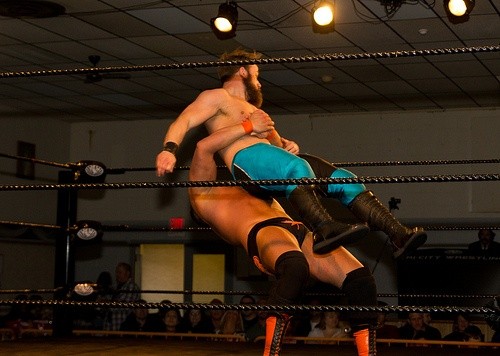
[240,119,253,134]
[163,141,179,155]
[267,130,277,141]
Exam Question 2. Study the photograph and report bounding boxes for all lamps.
[210,0,238,39]
[444,0,476,23]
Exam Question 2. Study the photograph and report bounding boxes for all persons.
[302,299,354,344]
[72,262,275,343]
[186,108,379,356]
[466,228,500,258]
[376,300,500,349]
[155,50,427,258]
[1,294,53,332]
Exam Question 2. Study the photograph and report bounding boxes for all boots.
[348,189,427,258]
[350,323,377,356]
[264,312,294,356]
[288,184,369,254]
